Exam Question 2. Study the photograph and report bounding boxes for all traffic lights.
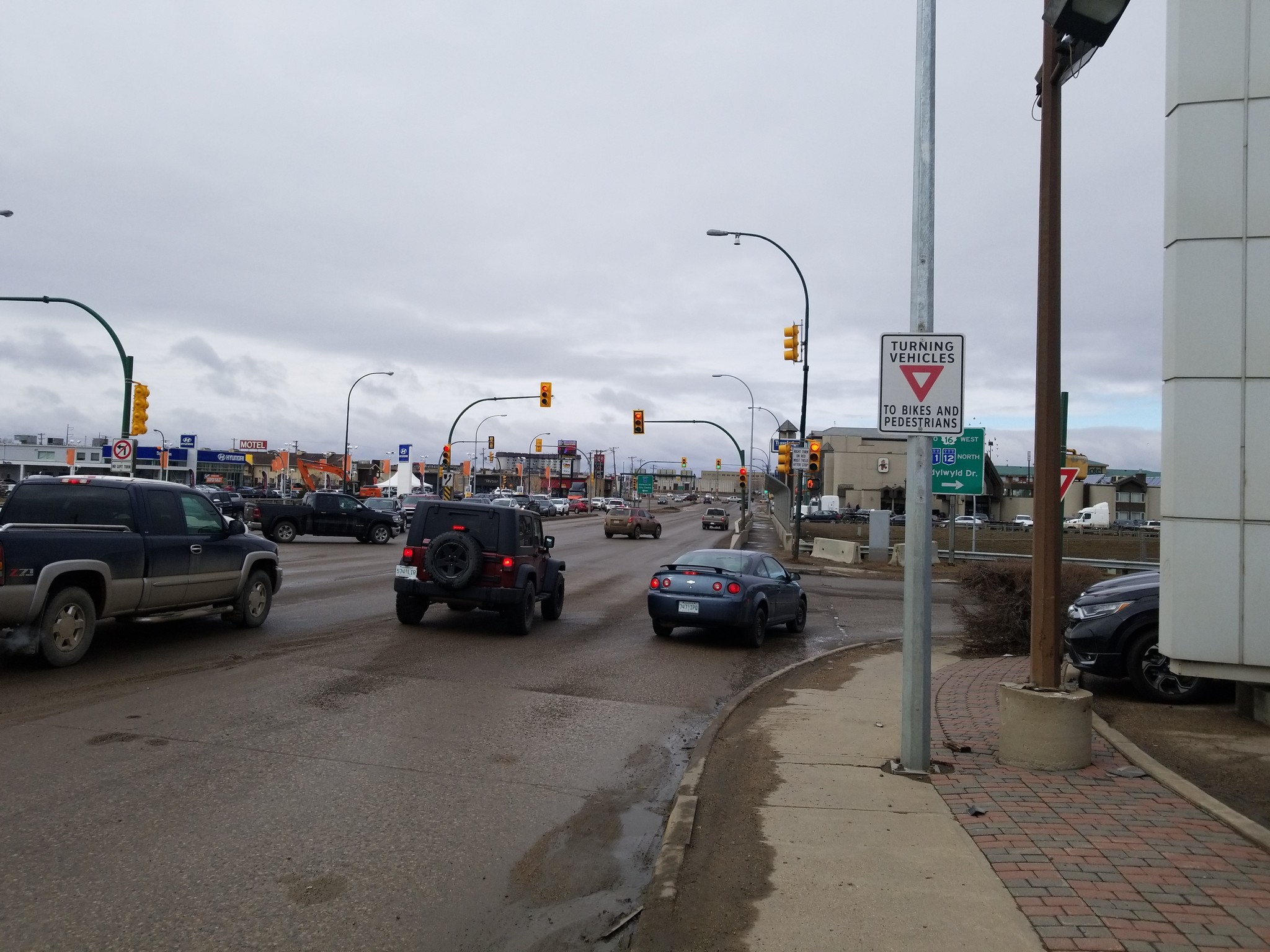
[777,441,821,472]
[633,410,645,434]
[807,477,821,490]
[764,489,768,495]
[540,382,552,407]
[489,451,494,462]
[633,477,636,489]
[783,324,799,361]
[739,467,747,488]
[503,475,507,484]
[716,458,721,469]
[131,383,150,435]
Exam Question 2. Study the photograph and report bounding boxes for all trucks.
[1064,501,1160,537]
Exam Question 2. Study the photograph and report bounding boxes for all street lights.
[989,439,994,521]
[343,371,394,495]
[706,228,811,560]
[712,374,754,518]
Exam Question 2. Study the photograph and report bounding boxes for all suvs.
[393,499,567,636]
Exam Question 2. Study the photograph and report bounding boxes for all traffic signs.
[932,427,986,496]
[637,475,653,494]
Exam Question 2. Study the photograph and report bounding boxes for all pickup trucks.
[0,472,285,669]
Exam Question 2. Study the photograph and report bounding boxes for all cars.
[1064,568,1208,705]
[503,485,506,489]
[647,549,807,648]
[152,406,781,543]
[770,490,991,529]
[1012,515,1034,531]
